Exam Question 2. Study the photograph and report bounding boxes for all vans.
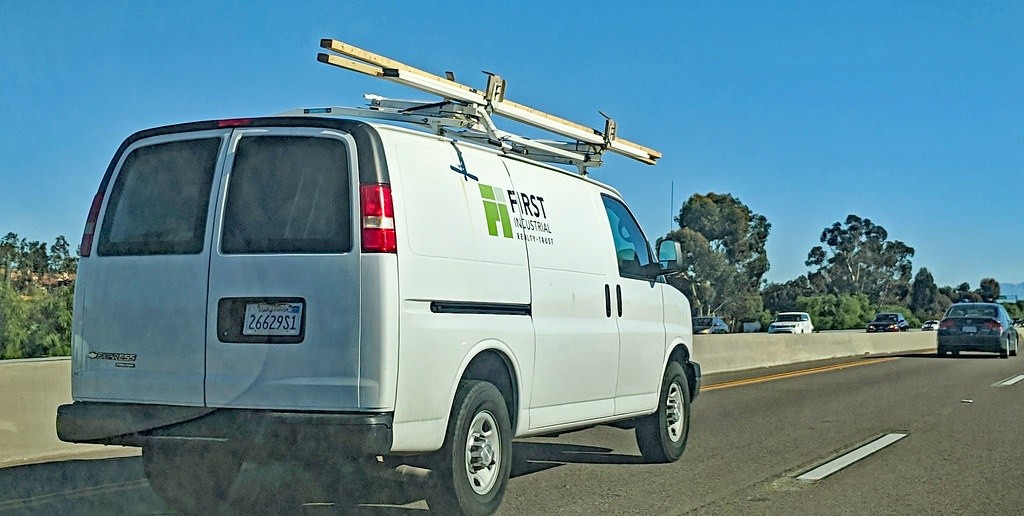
[55,105,703,516]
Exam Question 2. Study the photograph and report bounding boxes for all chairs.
[956,311,964,316]
[966,310,978,317]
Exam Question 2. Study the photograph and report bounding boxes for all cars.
[693,316,732,335]
[734,317,762,333]
[1014,321,1024,327]
[768,312,814,334]
[866,313,909,332]
[936,302,1019,359]
[921,320,939,331]
